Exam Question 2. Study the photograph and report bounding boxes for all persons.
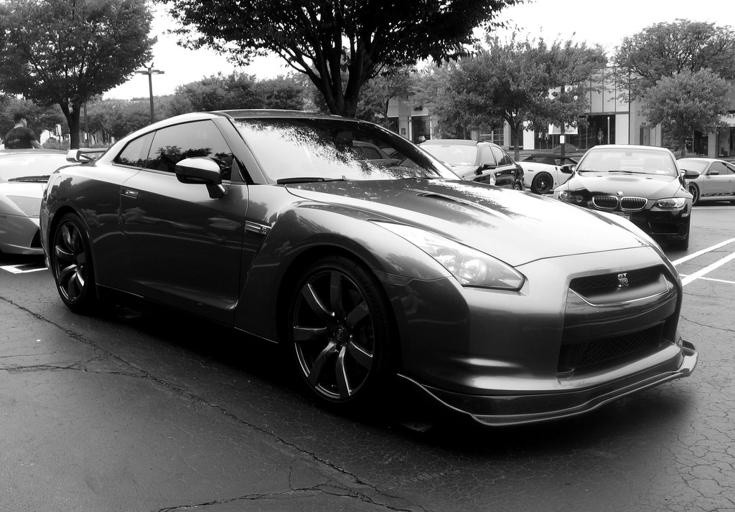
[721,146,728,157]
[4,113,42,149]
[597,128,604,144]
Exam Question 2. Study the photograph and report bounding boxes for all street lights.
[134,68,164,124]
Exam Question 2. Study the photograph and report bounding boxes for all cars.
[0,147,90,257]
[553,143,694,250]
[400,139,526,192]
[513,152,577,194]
[675,158,735,206]
[39,107,699,427]
